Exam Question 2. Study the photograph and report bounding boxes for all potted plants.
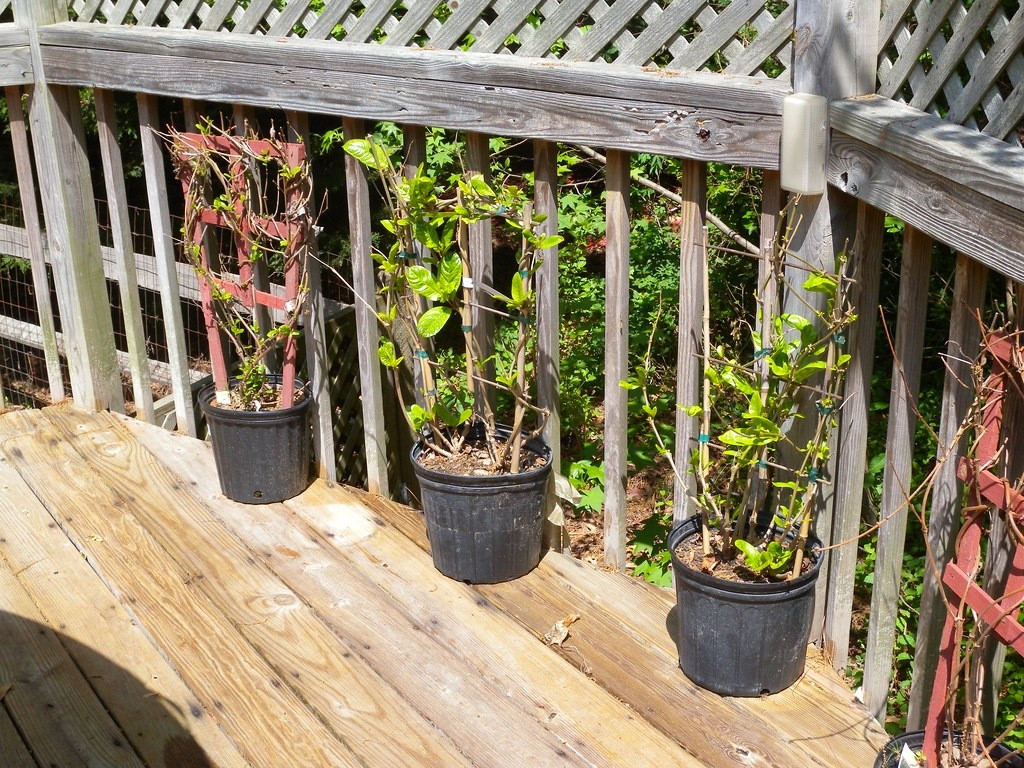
[151,111,333,502]
[607,191,860,700]
[338,135,570,582]
